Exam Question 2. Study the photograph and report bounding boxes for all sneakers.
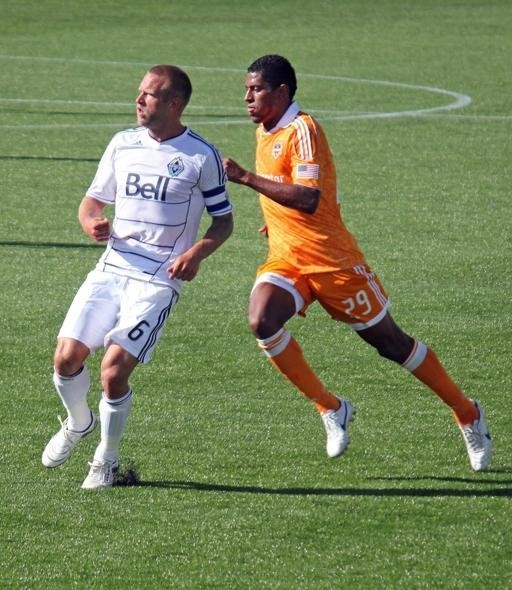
[41,411,98,468]
[457,398,491,471]
[319,396,355,459]
[80,449,120,490]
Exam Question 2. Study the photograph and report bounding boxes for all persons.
[41,65,234,490]
[222,55,492,472]
